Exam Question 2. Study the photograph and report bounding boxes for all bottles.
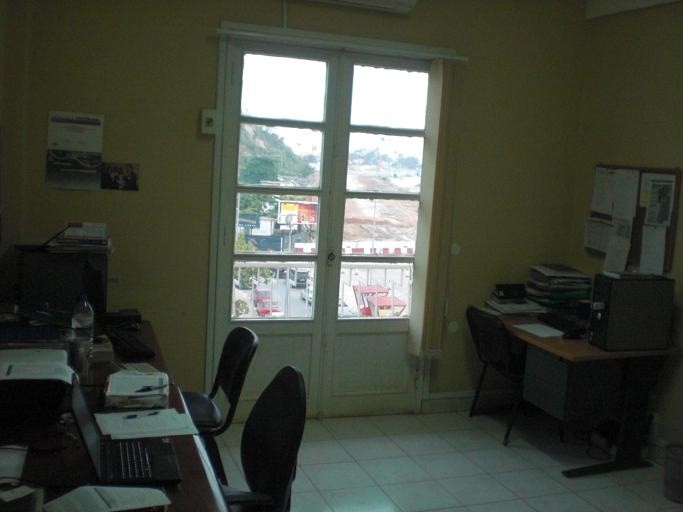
[71,294,93,362]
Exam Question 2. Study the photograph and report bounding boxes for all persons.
[103,165,137,189]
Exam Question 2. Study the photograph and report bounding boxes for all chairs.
[463,305,566,445]
[181,326,259,487]
[223,364,305,512]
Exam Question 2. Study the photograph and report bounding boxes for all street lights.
[369,195,377,254]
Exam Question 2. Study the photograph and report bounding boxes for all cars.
[233,262,314,319]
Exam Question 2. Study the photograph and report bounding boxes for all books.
[48,222,113,256]
[483,265,594,315]
[67,335,114,365]
[104,368,171,409]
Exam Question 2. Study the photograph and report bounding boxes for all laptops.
[68,373,183,486]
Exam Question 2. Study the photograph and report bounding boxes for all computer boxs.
[587,272,675,352]
[13,241,108,325]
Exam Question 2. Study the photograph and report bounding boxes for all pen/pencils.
[126,411,158,418]
[6,363,13,376]
[136,385,166,393]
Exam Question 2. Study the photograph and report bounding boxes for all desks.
[0,319,232,512]
[490,311,683,478]
